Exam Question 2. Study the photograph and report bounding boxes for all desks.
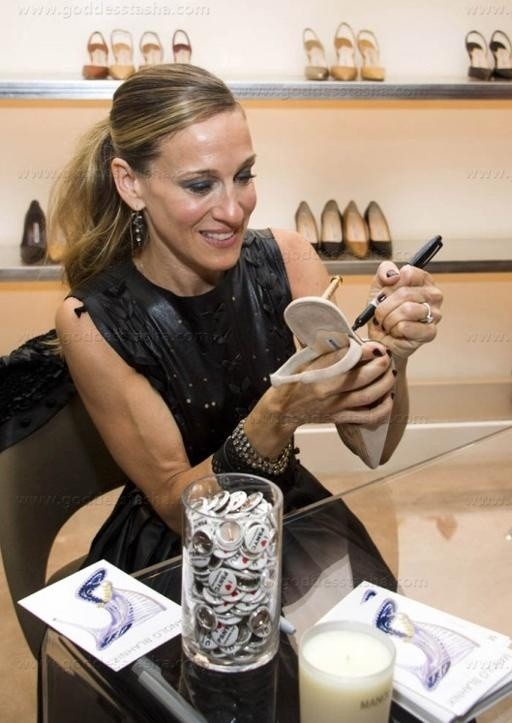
[31,423,512,723]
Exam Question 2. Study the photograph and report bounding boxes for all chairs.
[2,323,294,662]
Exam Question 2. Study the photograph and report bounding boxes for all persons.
[48,62,441,718]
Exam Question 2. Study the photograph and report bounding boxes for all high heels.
[82,31,111,80]
[332,21,358,82]
[490,30,512,80]
[20,199,49,266]
[172,28,191,64]
[357,29,386,81]
[302,27,330,82]
[270,274,397,470]
[364,201,394,260]
[108,28,135,80]
[343,201,369,260]
[296,201,319,254]
[139,31,163,72]
[320,200,344,260]
[464,30,490,80]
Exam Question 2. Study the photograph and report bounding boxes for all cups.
[180,473,284,673]
[298,620,396,723]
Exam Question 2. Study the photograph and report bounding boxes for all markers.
[348,232,442,335]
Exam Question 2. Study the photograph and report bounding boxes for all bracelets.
[231,418,298,476]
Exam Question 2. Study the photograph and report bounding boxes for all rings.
[424,300,434,325]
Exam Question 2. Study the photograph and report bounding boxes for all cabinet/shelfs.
[0,79,512,290]
